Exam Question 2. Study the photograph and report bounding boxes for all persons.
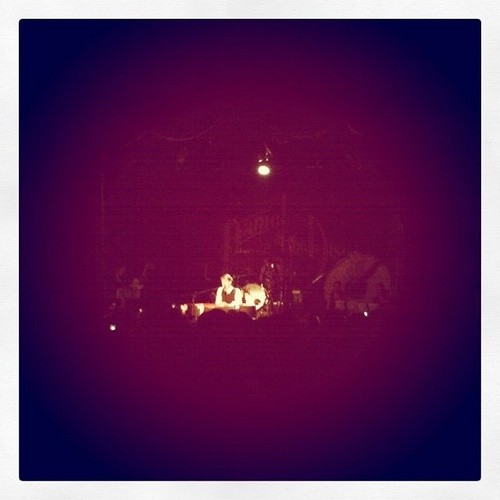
[216,273,244,306]
